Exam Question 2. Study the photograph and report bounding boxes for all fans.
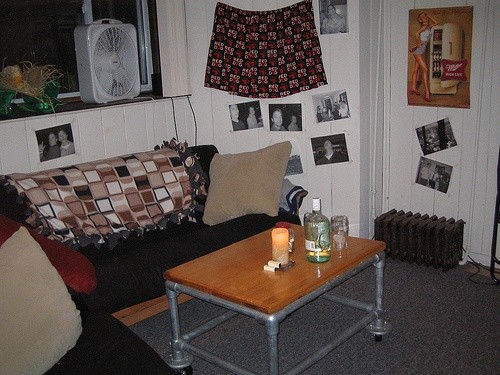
[74,19,141,105]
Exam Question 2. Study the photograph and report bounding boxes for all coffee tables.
[163,223,393,375]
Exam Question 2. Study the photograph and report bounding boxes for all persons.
[42,129,75,161]
[321,5,345,34]
[316,99,349,122]
[409,13,438,102]
[420,163,450,192]
[229,104,258,130]
[271,109,300,130]
[419,133,457,152]
[316,140,348,164]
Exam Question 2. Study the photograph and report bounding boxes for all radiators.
[374,208,466,273]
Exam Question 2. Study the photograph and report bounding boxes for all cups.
[0,89,16,115]
[330,216,349,252]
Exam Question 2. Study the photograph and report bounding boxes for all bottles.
[305,197,331,264]
[273,222,294,253]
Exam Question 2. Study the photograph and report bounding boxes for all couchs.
[0,136,309,375]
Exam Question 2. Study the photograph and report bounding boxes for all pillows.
[0,214,98,294]
[0,226,82,375]
[203,141,293,227]
